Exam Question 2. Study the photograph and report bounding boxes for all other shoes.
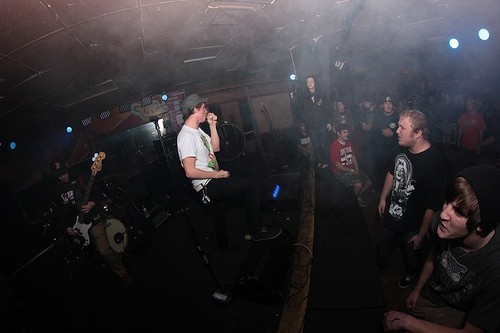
[251,226,282,243]
[398,272,416,289]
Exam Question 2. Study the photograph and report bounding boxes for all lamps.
[477,28,490,40]
[161,89,168,101]
[7,136,16,151]
[64,119,74,135]
[447,33,460,49]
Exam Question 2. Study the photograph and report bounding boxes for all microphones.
[206,115,228,124]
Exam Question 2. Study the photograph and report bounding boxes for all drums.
[90,218,129,260]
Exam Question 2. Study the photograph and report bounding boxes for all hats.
[455,164,500,238]
[381,95,394,103]
[48,157,67,177]
[180,94,208,116]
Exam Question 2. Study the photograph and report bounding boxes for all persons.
[303,74,500,333]
[51,162,136,285]
[177,94,283,252]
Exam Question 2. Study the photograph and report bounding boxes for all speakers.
[258,169,301,213]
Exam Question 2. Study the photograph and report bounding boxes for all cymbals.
[92,172,116,184]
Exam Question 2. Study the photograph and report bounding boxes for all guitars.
[64,149,107,248]
